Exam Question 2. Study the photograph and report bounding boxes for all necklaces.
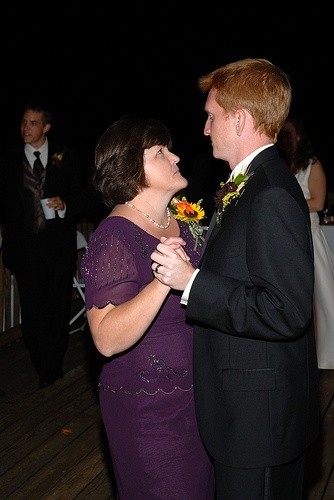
[124,201,171,229]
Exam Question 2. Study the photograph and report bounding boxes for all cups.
[40,198,56,220]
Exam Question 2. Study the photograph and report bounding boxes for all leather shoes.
[39,374,49,388]
[53,361,63,378]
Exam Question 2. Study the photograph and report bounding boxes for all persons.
[0,101,87,390]
[78,117,218,500]
[149,57,323,500]
[279,112,334,370]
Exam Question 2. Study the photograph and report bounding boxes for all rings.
[155,264,163,273]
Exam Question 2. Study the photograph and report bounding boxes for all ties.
[32,151,44,180]
[200,209,217,262]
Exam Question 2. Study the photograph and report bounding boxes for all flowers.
[212,173,249,223]
[169,195,209,251]
